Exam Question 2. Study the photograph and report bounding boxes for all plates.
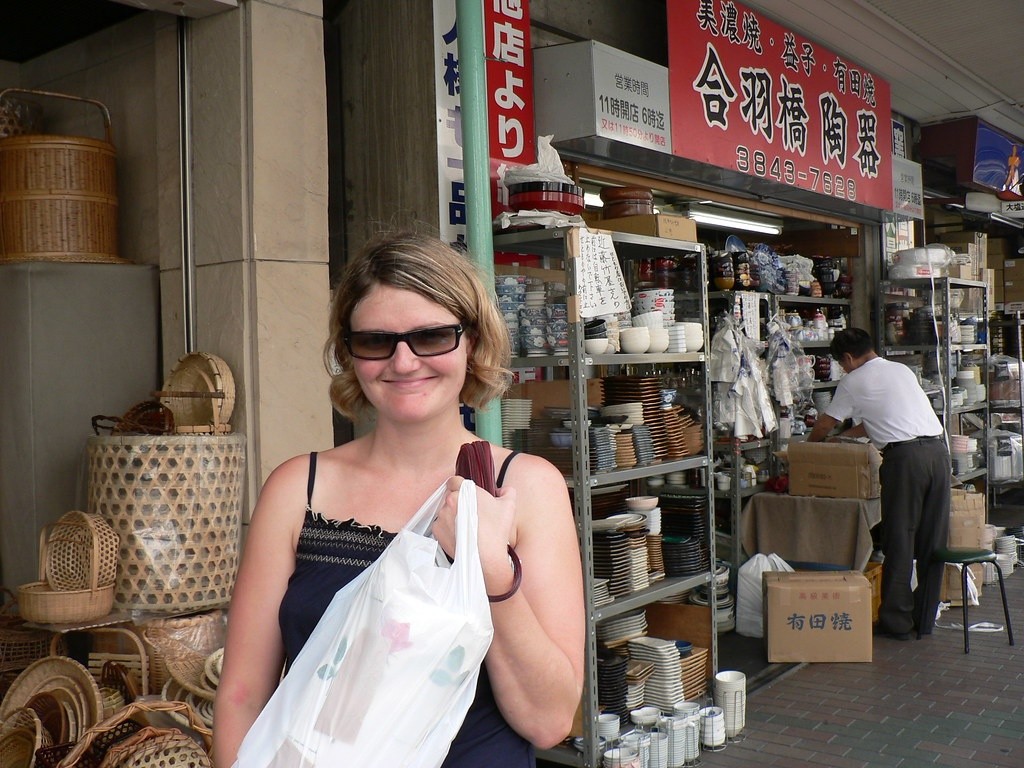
[499,373,705,489]
[559,492,748,768]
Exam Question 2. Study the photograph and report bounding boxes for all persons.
[807,325,952,640]
[213,229,585,768]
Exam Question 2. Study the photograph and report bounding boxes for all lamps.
[923,187,965,209]
[682,202,784,236]
[988,211,1024,229]
[585,188,661,214]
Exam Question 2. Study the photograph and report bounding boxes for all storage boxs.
[946,241,976,275]
[1004,302,1024,315]
[987,255,1005,269]
[592,214,699,243]
[995,303,1004,311]
[939,231,980,275]
[770,439,882,501]
[948,485,993,547]
[950,264,971,280]
[980,232,988,268]
[995,287,1004,303]
[1003,258,1024,281]
[760,570,875,664]
[987,238,1006,255]
[1004,281,1024,302]
[946,561,988,602]
[994,270,1004,287]
[861,560,885,624]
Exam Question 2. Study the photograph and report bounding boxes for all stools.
[914,546,1015,654]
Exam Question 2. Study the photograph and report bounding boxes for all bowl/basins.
[709,244,854,300]
[977,523,1024,586]
[489,253,708,358]
[885,301,988,479]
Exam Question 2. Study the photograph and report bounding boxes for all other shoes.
[873,621,909,640]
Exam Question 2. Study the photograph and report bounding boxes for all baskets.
[1,349,235,768]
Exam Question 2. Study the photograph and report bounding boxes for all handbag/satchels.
[232,480,495,768]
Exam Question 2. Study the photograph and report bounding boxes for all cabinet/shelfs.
[490,224,729,768]
[770,293,852,483]
[878,273,991,524]
[669,286,777,572]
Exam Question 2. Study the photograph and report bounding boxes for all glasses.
[337,323,472,365]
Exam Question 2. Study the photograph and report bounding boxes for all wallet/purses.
[456,441,496,497]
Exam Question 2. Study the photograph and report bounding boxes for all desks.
[744,488,887,572]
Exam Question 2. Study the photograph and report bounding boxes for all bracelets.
[486,544,523,603]
